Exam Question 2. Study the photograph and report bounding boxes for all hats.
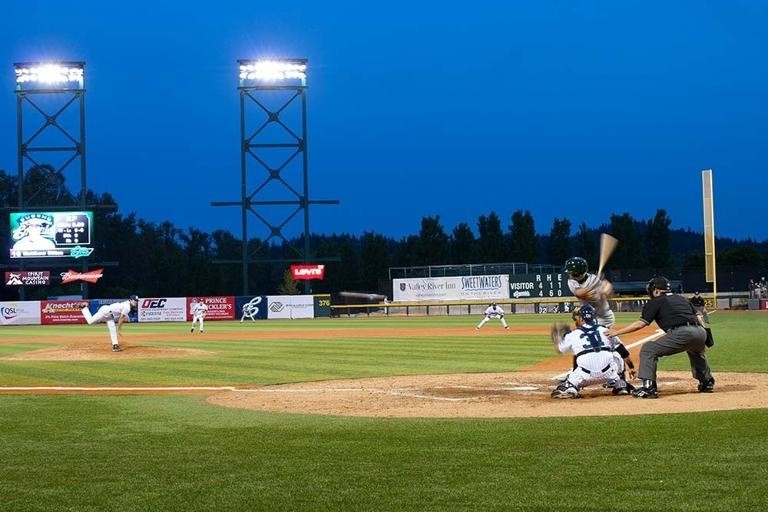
[131,295,141,301]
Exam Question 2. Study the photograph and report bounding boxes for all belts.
[671,322,701,329]
[582,365,610,374]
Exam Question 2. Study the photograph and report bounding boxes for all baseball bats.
[595,232,619,281]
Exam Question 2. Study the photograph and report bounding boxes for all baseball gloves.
[552,323,569,345]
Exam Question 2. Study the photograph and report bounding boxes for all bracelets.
[627,362,635,369]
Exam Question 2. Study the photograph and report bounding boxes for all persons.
[384,296,389,313]
[563,257,616,388]
[603,276,715,398]
[748,277,768,299]
[240,305,255,322]
[12,220,55,249]
[79,295,139,351]
[191,299,208,333]
[551,304,635,398]
[476,303,509,330]
[539,293,622,312]
[690,291,709,323]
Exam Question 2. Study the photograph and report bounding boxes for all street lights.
[232,57,316,294]
[11,59,97,300]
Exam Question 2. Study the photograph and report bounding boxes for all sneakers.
[112,344,120,352]
[551,377,715,398]
[73,304,85,310]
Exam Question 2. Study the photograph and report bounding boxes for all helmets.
[564,257,588,278]
[646,277,674,298]
[572,303,598,326]
